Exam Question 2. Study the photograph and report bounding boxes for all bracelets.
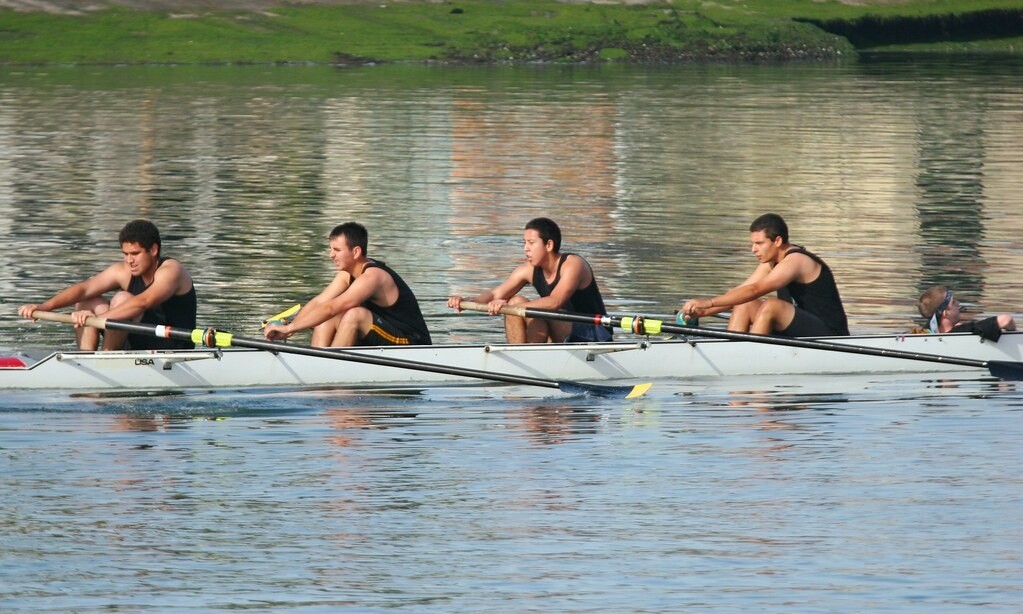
[709,299,713,307]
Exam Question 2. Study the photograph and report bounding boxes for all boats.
[0,364,1000,404]
[0,319,1023,390]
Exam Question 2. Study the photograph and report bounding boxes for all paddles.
[460,300,1023,381]
[606,308,698,325]
[261,302,302,338]
[33,309,653,401]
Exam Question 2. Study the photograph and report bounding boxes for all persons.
[682,213,851,337]
[447,217,614,344]
[265,222,432,346]
[18,219,197,351]
[918,287,1017,343]
